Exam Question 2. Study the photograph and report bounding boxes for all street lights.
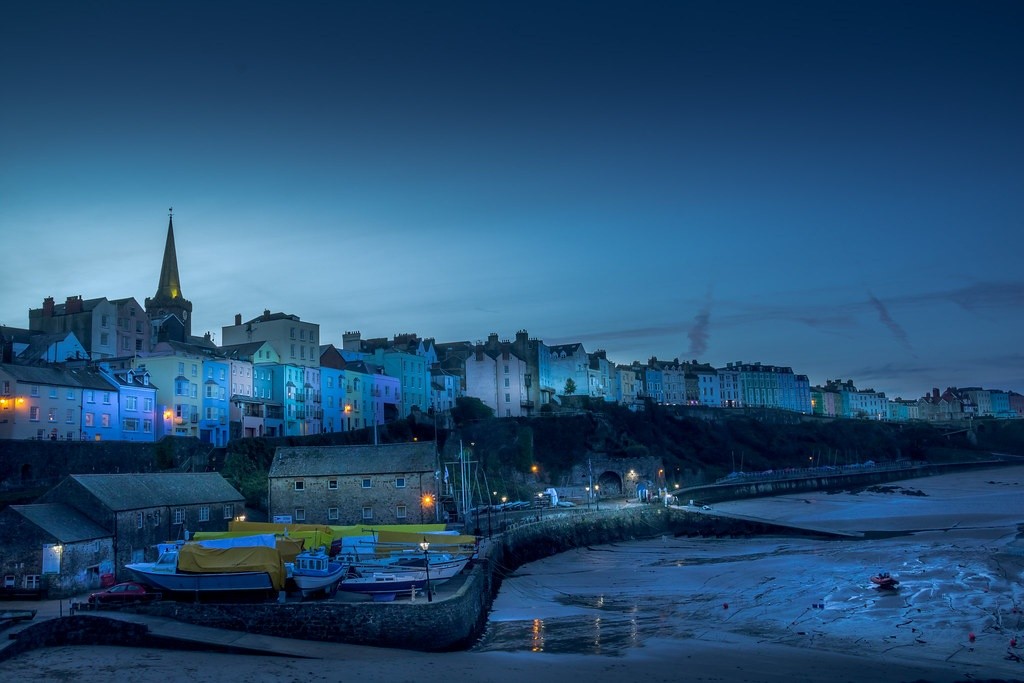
[493,491,497,514]
[53,542,63,617]
[502,497,507,530]
[538,493,543,520]
[595,484,599,510]
[419,536,432,602]
[586,487,590,508]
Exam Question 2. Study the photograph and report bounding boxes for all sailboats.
[808,448,840,474]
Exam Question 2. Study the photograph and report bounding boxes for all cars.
[702,505,711,510]
[88,581,164,608]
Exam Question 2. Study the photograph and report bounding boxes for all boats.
[870,576,899,587]
[126,532,470,596]
